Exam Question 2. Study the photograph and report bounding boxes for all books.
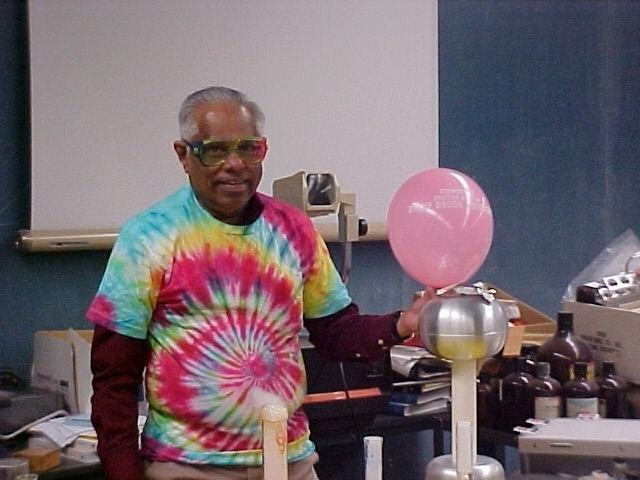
[385,345,451,418]
[27,411,146,464]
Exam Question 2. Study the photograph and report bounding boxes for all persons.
[82,82,437,479]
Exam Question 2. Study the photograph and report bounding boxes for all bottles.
[477,310,630,435]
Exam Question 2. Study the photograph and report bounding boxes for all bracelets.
[392,311,415,344]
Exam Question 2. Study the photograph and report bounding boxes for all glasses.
[182,137,268,166]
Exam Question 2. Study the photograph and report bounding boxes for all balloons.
[385,164,496,293]
